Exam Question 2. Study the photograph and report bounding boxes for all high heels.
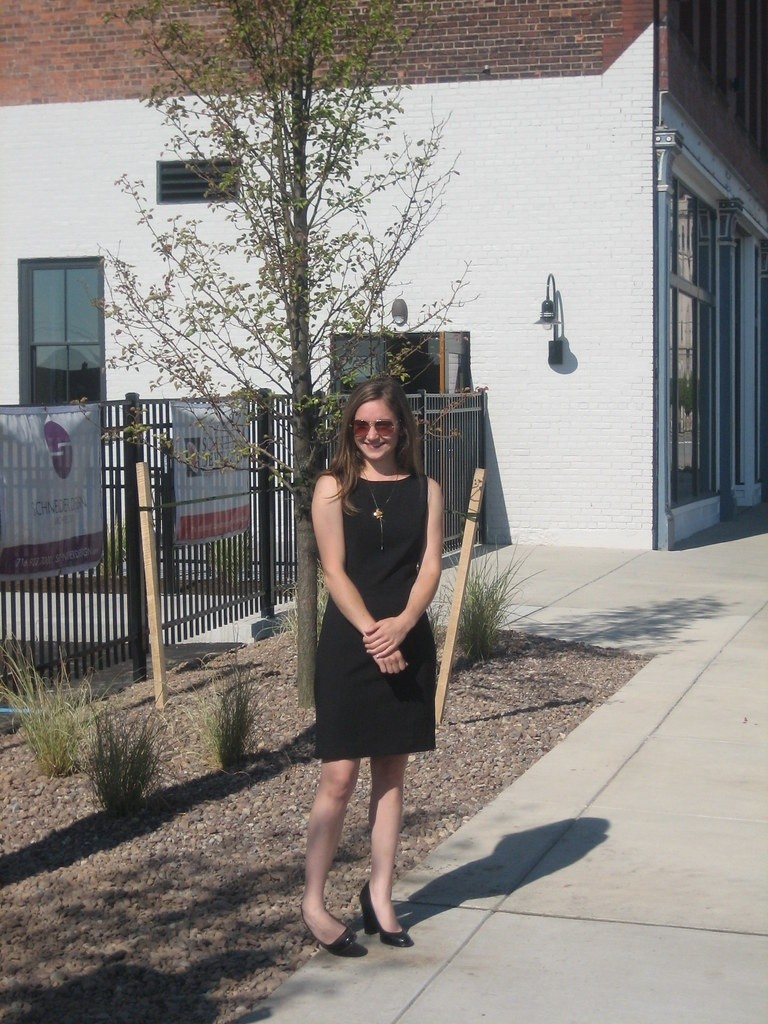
[298,904,357,951]
[360,881,412,948]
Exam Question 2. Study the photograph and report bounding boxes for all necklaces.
[361,468,398,551]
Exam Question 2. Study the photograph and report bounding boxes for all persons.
[300,379,443,954]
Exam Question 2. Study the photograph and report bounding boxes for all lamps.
[533,273,565,366]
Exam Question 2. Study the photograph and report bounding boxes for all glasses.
[352,419,401,436]
